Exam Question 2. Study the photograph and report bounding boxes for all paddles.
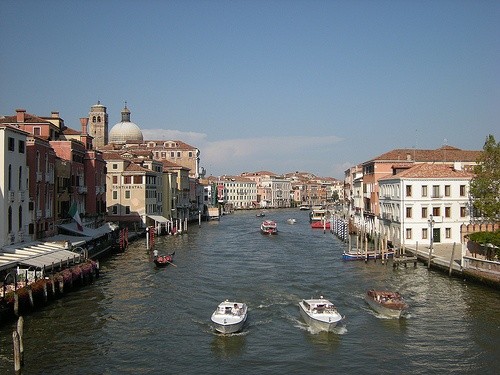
[167,261,177,267]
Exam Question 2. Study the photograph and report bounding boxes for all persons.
[153,249,158,257]
[231,304,239,314]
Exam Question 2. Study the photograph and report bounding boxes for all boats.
[310,209,332,228]
[211,299,249,334]
[342,248,396,260]
[256,212,265,217]
[261,220,277,234]
[300,204,323,210]
[154,251,176,266]
[298,298,342,332]
[366,288,404,319]
